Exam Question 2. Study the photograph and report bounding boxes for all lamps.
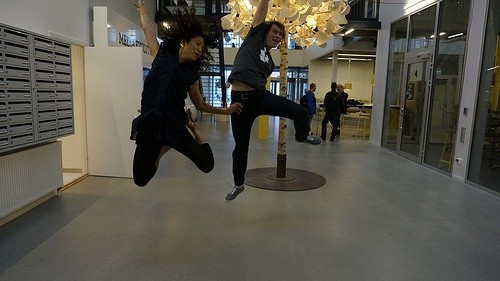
[221,0,351,191]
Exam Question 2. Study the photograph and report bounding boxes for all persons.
[130,0,243,188]
[305,82,317,136]
[331,84,349,135]
[319,81,342,141]
[224,0,323,202]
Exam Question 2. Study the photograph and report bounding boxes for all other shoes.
[186,108,197,128]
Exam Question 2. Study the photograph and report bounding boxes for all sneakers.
[226,185,244,199]
[304,136,321,145]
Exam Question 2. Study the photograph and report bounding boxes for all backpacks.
[300,92,312,108]
[329,91,342,114]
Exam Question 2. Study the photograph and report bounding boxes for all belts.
[231,90,262,101]
